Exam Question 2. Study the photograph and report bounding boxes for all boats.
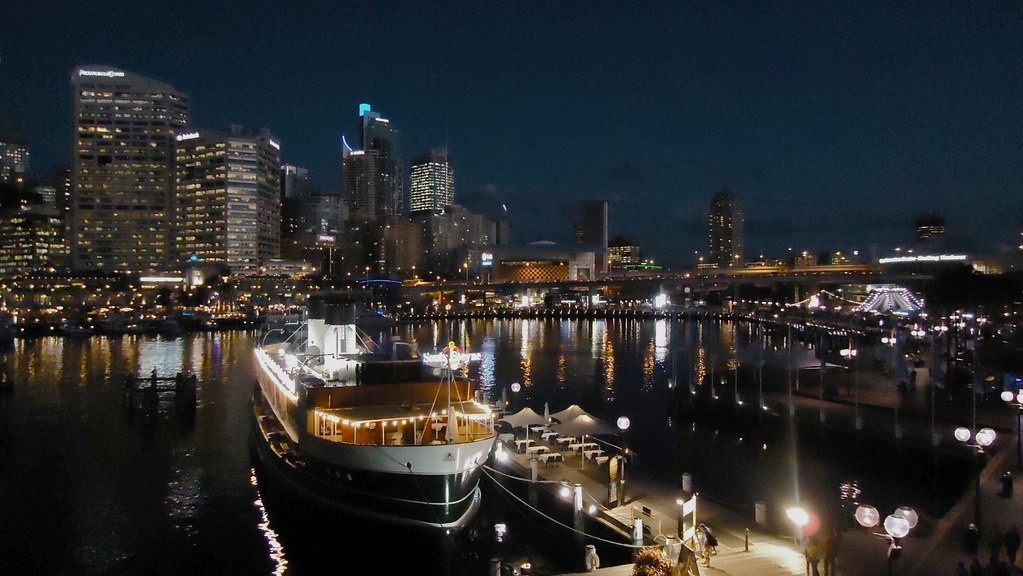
[248,252,497,538]
[59,323,91,338]
[0,361,15,391]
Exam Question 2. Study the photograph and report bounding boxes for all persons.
[956,525,1023,576]
[698,523,712,568]
[805,530,842,576]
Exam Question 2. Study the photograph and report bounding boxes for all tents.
[445,406,459,444]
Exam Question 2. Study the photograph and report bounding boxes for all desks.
[584,450,604,460]
[514,440,534,454]
[538,453,565,466]
[596,456,623,466]
[499,433,519,444]
[569,442,599,454]
[531,427,547,431]
[526,447,550,458]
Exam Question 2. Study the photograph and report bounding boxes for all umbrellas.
[497,409,555,447]
[548,405,608,442]
[549,415,617,468]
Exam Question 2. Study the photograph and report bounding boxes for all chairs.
[514,429,622,470]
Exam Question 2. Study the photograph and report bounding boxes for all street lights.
[1001,389,1023,467]
[954,426,996,530]
[881,337,896,378]
[855,503,918,576]
[617,417,630,505]
